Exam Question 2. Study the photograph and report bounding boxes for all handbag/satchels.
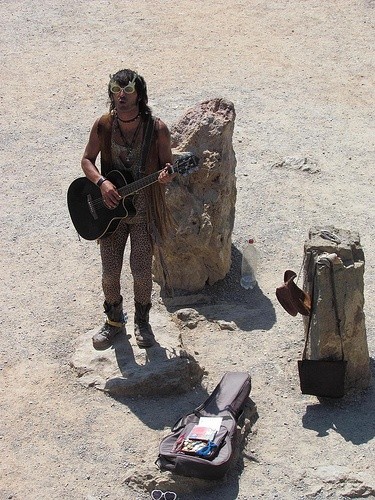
[298,360,347,398]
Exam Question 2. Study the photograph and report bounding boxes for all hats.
[276,270,311,316]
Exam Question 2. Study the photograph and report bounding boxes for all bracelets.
[96,177,109,188]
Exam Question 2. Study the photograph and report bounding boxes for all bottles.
[239,239,257,289]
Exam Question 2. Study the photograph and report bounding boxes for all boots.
[134,298,155,347]
[91,295,127,350]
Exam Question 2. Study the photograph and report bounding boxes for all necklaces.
[115,110,142,122]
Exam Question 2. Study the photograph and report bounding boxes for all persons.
[80,69,175,347]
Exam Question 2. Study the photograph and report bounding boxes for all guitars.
[67,154,201,241]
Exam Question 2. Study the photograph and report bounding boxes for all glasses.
[151,490,177,500]
[109,73,137,94]
